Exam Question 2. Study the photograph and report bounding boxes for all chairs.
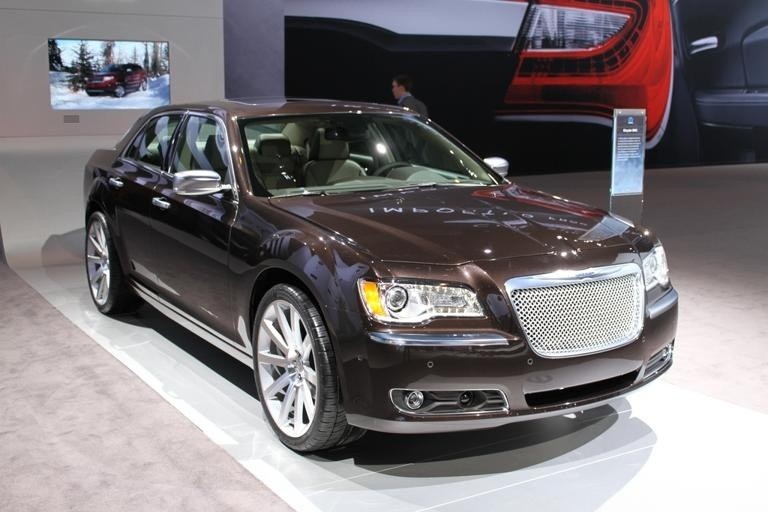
[206,131,370,190]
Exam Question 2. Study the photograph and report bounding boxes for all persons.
[391,73,434,168]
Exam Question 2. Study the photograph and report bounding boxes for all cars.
[82,97,681,454]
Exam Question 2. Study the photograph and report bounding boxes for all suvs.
[84,63,147,97]
